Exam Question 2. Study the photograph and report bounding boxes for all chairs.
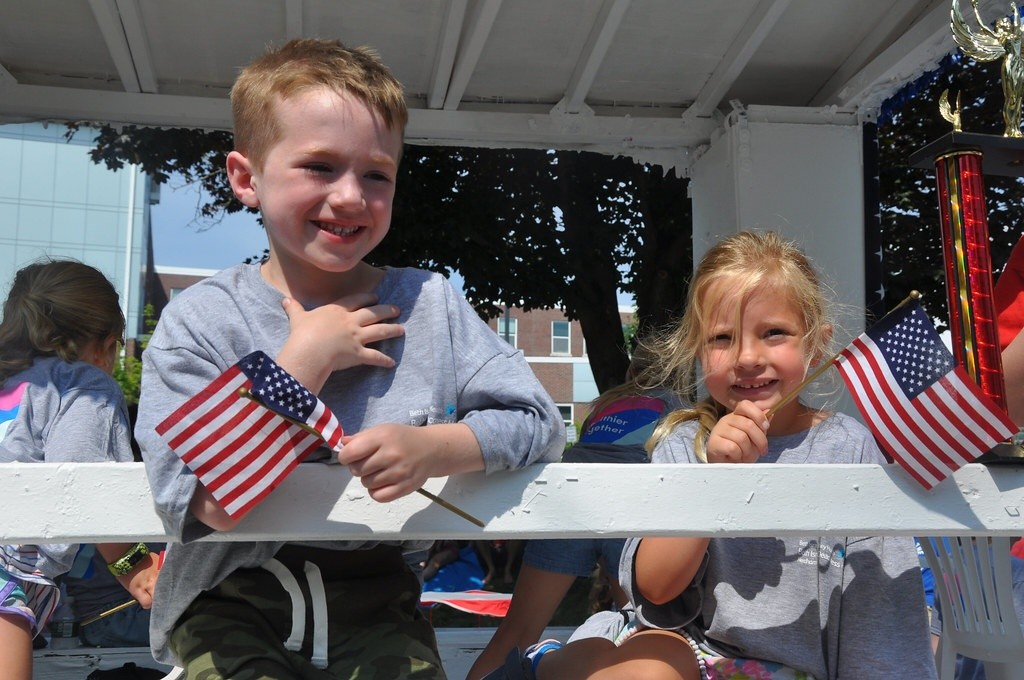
[917,536,1024,680]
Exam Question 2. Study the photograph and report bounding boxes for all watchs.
[107,543,150,578]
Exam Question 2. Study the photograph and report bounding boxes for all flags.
[157,346,347,521]
[833,301,1017,491]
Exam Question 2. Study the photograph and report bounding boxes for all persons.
[474,230,937,680]
[938,536,1023,680]
[992,232,1024,430]
[0,261,164,680]
[915,537,952,657]
[466,332,698,680]
[132,38,565,680]
